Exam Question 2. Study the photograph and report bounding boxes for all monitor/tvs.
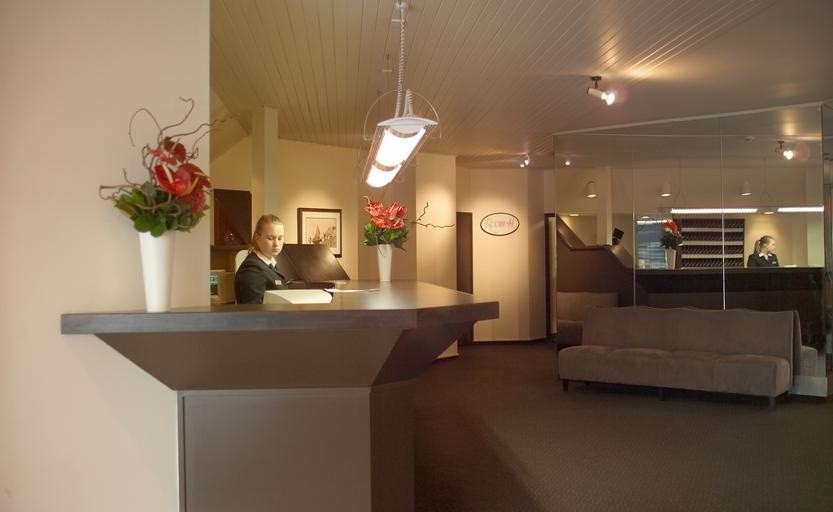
[262,288,332,305]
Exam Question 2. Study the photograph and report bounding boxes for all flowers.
[98,90,235,234]
[362,195,458,253]
[660,219,685,250]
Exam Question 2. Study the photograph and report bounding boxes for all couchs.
[558,305,796,409]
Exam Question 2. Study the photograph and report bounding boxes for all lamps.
[584,179,754,199]
[587,76,616,105]
[363,2,435,191]
[774,140,794,161]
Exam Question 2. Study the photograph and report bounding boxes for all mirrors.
[551,105,824,360]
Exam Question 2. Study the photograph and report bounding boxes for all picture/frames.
[297,206,343,256]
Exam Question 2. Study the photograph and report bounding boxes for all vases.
[137,224,181,313]
[376,241,393,282]
[665,248,676,270]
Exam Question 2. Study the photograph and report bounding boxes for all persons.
[747,235,779,268]
[232,213,291,303]
